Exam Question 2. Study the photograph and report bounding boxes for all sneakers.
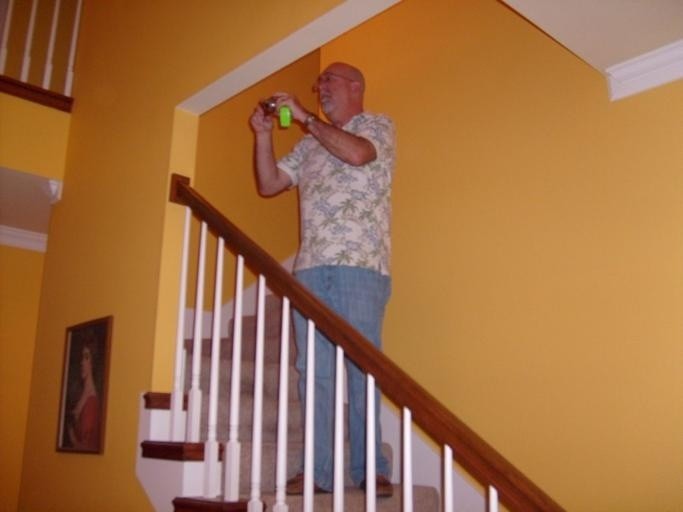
[286,473,317,495]
[376,475,393,497]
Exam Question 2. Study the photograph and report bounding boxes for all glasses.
[311,70,355,92]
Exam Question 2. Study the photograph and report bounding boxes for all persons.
[62,327,105,454]
[248,59,399,499]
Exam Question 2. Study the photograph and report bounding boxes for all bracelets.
[299,107,319,130]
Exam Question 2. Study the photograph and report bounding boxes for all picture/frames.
[55,314,113,454]
